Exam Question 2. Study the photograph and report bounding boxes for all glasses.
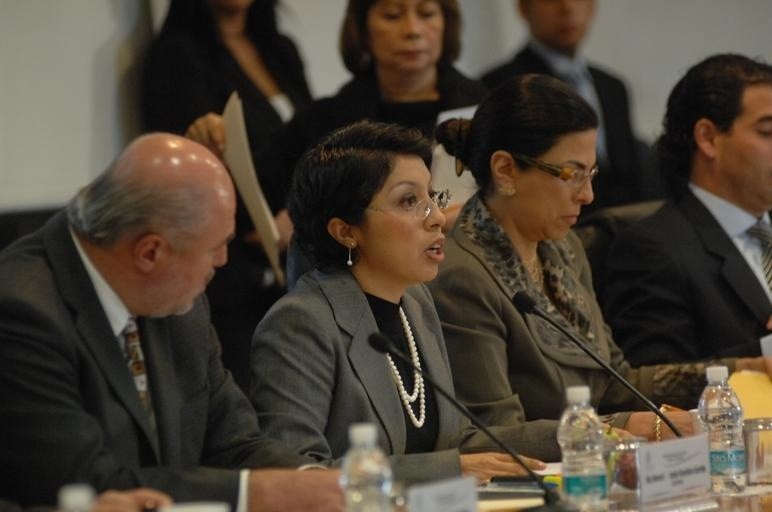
[367,187,452,220]
[514,151,600,188]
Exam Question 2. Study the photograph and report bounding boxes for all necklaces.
[519,253,544,294]
[372,305,426,429]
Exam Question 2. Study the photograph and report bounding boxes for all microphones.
[368,331,577,511]
[510,287,679,438]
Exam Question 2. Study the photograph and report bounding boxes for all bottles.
[339,422,393,511]
[699,365,747,492]
[556,386,610,512]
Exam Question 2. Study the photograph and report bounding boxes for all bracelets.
[655,404,665,450]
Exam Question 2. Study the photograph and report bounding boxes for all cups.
[616,437,646,489]
[742,416,771,487]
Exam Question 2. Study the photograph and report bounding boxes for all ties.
[122,318,158,437]
[747,222,772,294]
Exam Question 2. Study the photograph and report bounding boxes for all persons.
[1,130,356,512]
[426,73,770,464]
[470,0,670,219]
[2,476,174,511]
[130,0,313,361]
[601,52,770,366]
[249,119,650,489]
[272,0,488,175]
